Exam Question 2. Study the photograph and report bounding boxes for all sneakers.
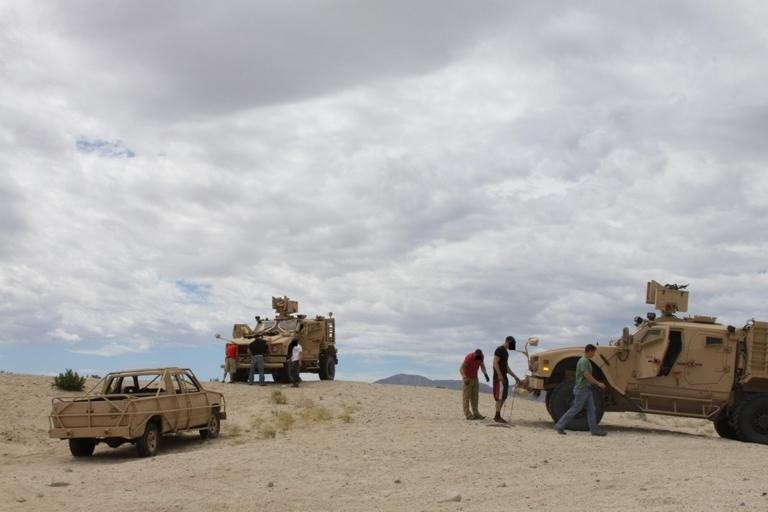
[592,431,607,436]
[494,416,506,423]
[466,414,484,419]
[554,425,566,434]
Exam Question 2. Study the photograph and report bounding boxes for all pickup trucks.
[50,367,228,459]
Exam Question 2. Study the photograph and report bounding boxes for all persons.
[459,349,489,419]
[287,338,302,387]
[248,333,269,386]
[553,344,607,436]
[221,342,239,383]
[493,335,522,423]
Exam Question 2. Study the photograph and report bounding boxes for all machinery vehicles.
[212,294,339,383]
[504,279,767,443]
